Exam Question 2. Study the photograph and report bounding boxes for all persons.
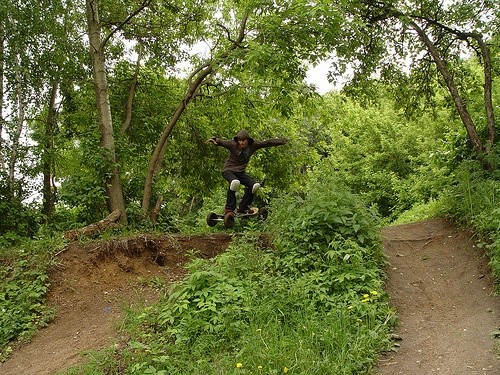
[205,129,288,216]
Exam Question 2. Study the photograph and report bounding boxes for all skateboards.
[206,206,268,228]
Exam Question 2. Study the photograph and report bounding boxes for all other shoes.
[225,209,233,215]
[237,206,254,213]
